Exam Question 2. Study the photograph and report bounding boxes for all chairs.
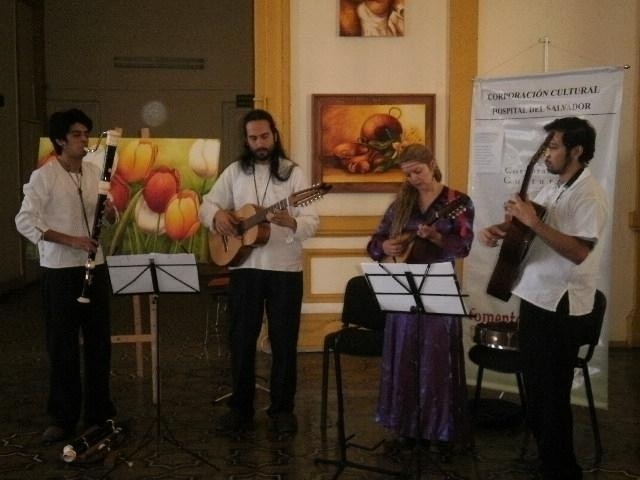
[321,275,388,466]
[469,288,606,466]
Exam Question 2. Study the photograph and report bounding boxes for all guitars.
[209,183,332,268]
[486,130,555,302]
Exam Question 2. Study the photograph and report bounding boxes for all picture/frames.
[310,93,437,193]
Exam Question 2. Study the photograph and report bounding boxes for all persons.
[195,106,321,422]
[365,141,475,456]
[13,107,120,444]
[473,116,610,479]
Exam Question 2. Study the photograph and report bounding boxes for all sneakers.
[215,412,253,431]
[511,457,583,479]
[383,435,415,452]
[429,439,447,453]
[42,425,73,440]
[277,417,297,437]
